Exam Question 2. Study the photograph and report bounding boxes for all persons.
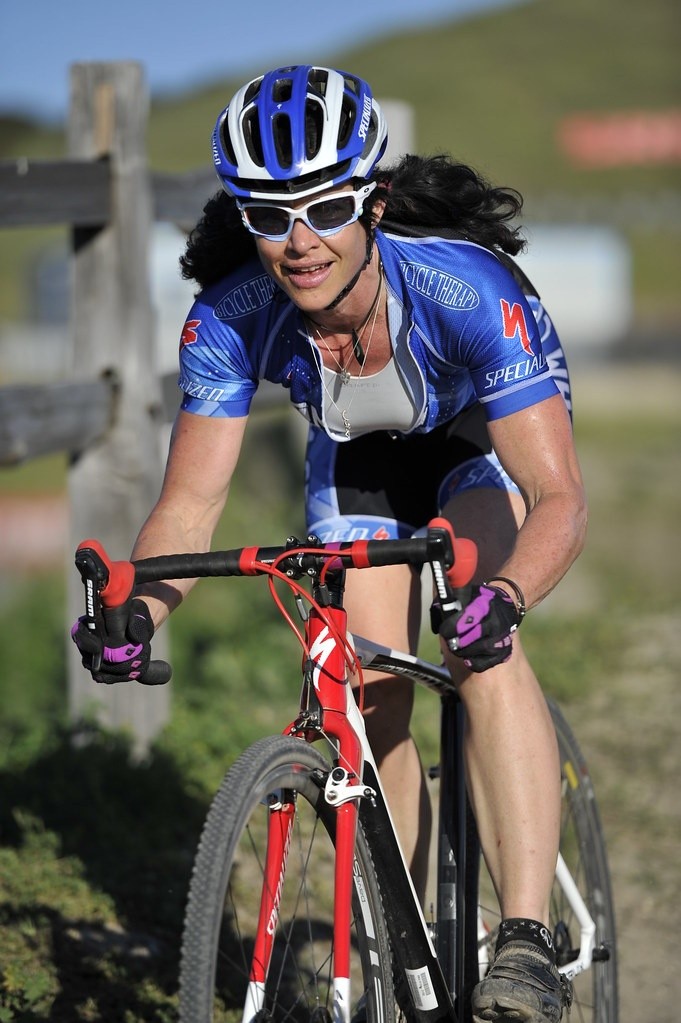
[71,67,590,1023]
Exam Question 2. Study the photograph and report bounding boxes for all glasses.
[235,181,378,242]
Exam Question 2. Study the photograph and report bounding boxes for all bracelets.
[482,576,526,625]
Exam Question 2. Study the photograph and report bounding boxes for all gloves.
[431,583,519,673]
[70,598,154,683]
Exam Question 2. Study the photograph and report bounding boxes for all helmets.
[210,65,388,205]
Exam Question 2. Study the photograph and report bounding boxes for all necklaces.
[306,266,383,440]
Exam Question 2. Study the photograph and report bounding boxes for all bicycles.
[74,516,619,1023]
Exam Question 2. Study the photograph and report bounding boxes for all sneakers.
[469,939,575,1022]
